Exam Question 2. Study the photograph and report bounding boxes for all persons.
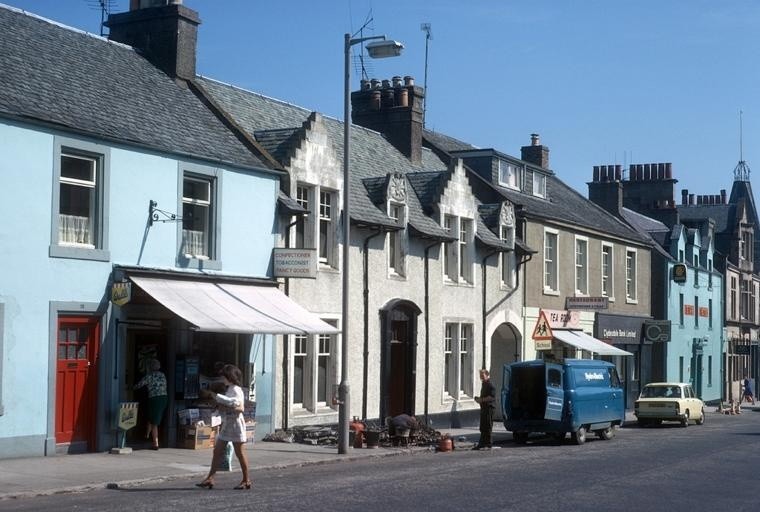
[669,387,679,397]
[740,375,755,405]
[472,368,497,449]
[195,363,252,490]
[134,359,168,450]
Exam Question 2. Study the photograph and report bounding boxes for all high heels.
[234,480,252,489]
[195,480,215,490]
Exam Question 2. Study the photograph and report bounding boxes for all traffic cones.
[716,399,742,416]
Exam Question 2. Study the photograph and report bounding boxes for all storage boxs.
[176,374,258,450]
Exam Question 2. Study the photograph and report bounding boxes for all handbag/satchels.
[215,433,234,472]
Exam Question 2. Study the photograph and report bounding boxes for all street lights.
[338,31,405,456]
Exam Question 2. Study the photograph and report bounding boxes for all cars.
[633,381,706,428]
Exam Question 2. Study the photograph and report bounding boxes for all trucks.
[500,357,626,445]
